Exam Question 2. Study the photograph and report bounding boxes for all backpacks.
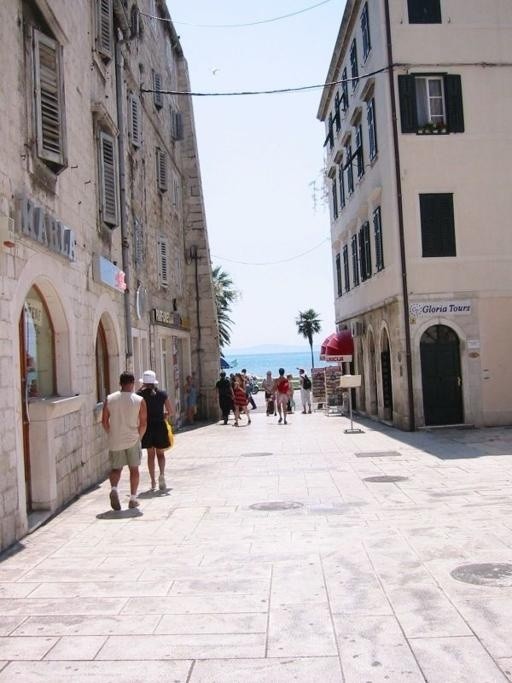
[301,374,311,389]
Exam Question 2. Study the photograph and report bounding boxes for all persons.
[213,369,312,427]
[182,375,198,425]
[136,370,174,491]
[101,371,147,510]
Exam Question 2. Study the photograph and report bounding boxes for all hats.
[139,370,159,385]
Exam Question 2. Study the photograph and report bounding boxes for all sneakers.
[109,490,121,511]
[128,499,139,508]
[158,475,167,490]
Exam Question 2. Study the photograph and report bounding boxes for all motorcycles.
[250,376,259,394]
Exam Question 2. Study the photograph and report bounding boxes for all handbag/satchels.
[164,418,174,450]
